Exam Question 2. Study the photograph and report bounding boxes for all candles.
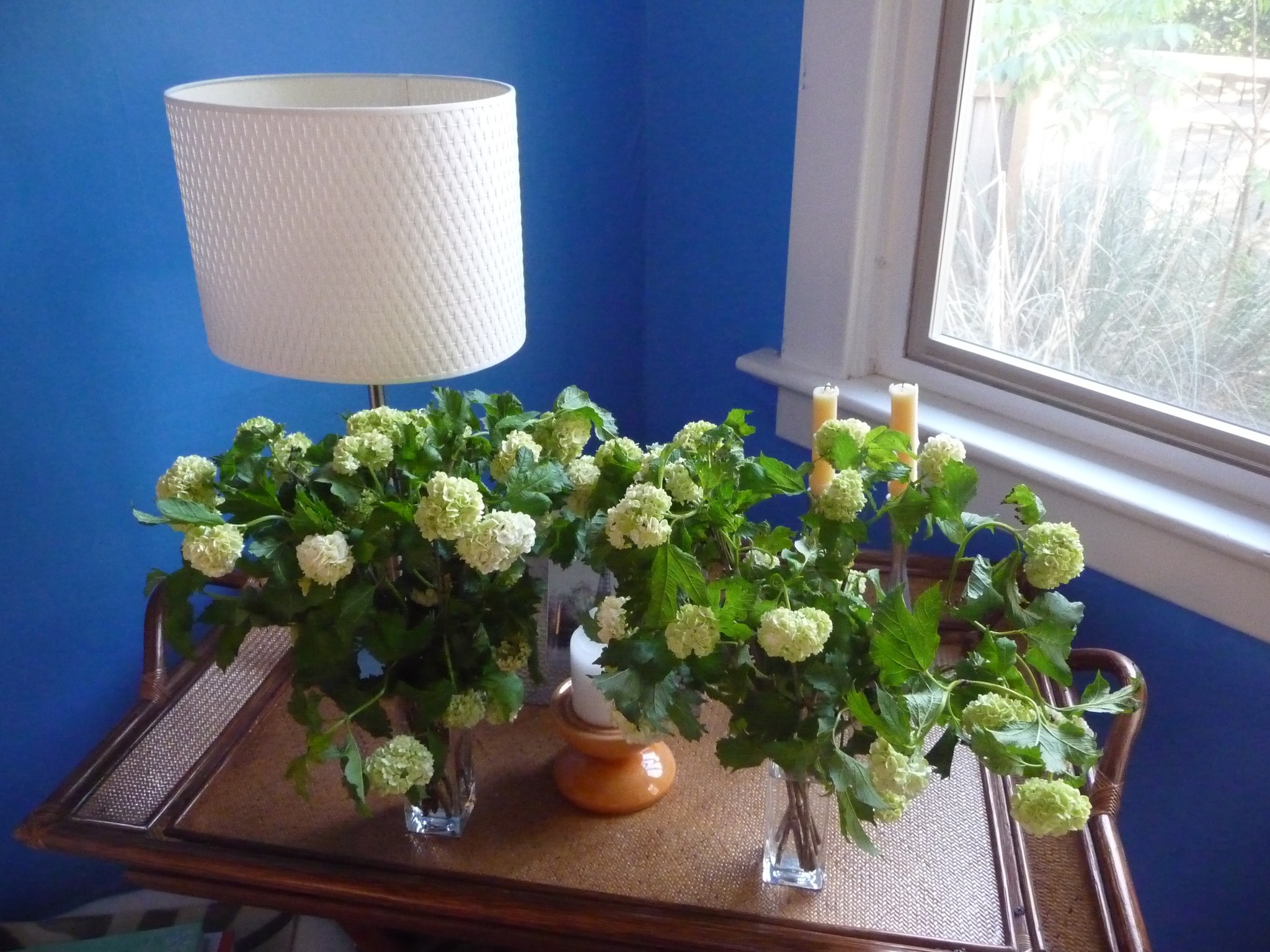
[565,621,630,731]
[887,378,921,500]
[809,382,840,501]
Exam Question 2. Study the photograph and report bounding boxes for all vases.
[544,537,620,692]
[387,681,477,837]
[755,757,834,894]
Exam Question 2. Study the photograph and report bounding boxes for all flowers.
[126,381,1147,875]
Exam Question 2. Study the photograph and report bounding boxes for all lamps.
[160,70,529,420]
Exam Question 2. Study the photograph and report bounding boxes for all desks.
[15,547,1152,952]
